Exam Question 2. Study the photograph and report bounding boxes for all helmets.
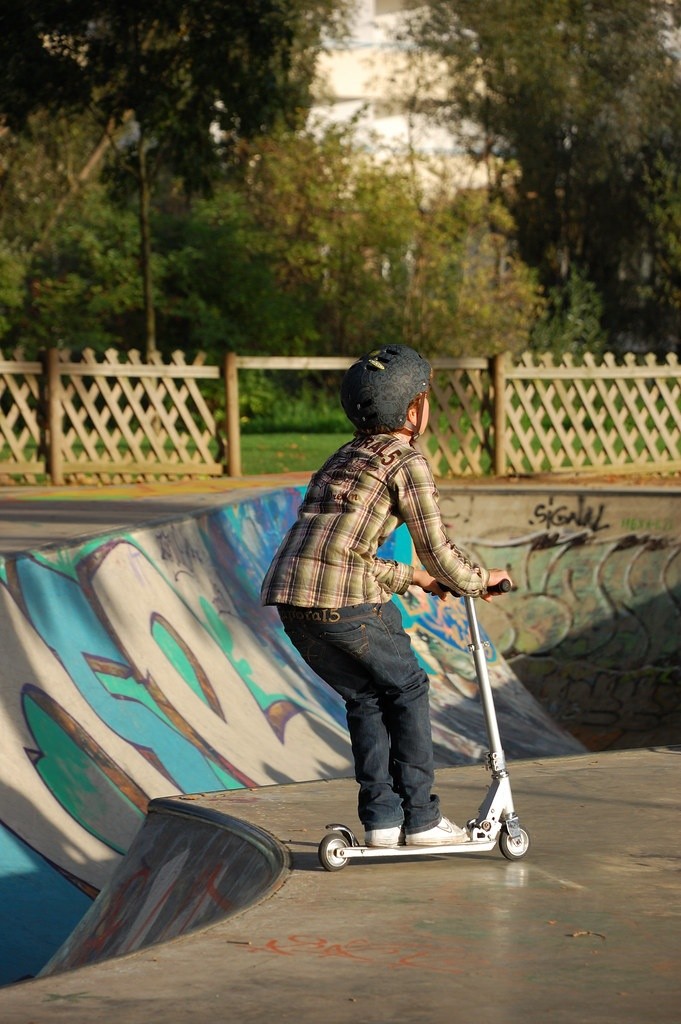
[339,343,431,435]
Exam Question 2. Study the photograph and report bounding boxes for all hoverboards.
[314,579,529,870]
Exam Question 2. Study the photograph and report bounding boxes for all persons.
[258,342,515,847]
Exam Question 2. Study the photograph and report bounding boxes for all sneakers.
[363,817,467,844]
